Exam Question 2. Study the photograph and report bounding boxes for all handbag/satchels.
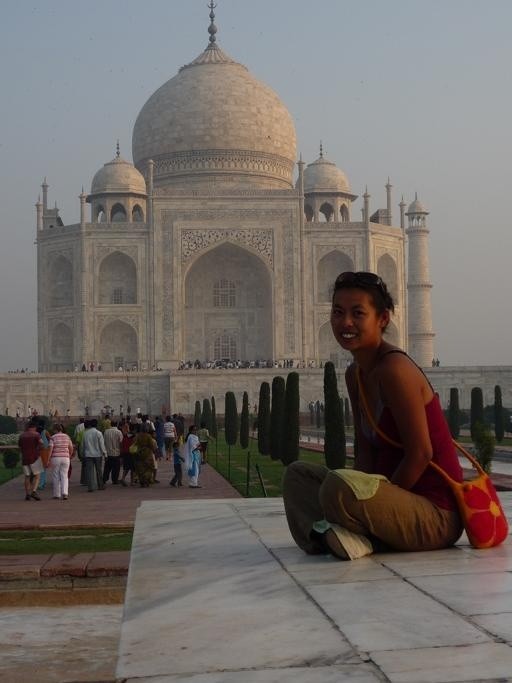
[454,472,508,548]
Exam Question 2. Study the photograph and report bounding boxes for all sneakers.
[31,492,40,500]
[26,495,30,499]
[325,528,373,560]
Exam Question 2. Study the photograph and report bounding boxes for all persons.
[432,358,436,367]
[436,359,440,367]
[282,272,465,560]
[5,404,214,501]
